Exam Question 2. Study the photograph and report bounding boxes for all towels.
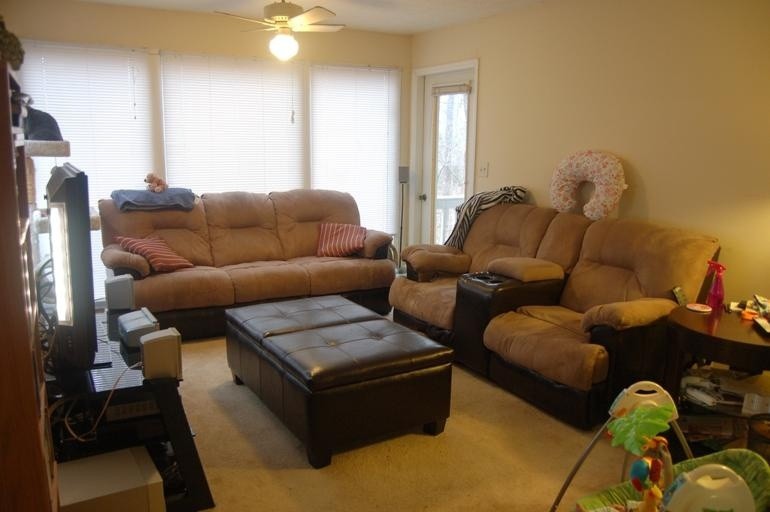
[110,188,196,212]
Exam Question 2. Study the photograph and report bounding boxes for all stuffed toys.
[143,172,166,194]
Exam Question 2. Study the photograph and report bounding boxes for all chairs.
[389,203,556,366]
[472,218,723,431]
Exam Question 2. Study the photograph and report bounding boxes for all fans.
[214,0,344,32]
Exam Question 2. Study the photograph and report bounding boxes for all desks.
[665,302,770,460]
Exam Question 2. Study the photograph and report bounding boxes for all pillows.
[115,232,196,272]
[317,223,365,257]
[549,151,630,219]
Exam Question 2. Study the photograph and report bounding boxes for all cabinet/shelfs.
[47,310,217,512]
[0,57,59,512]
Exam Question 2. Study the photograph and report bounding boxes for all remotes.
[752,316,770,335]
[686,387,716,407]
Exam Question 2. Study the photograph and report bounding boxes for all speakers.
[138,325,184,381]
[104,273,137,312]
[116,306,160,347]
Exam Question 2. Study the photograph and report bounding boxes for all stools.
[224,294,456,469]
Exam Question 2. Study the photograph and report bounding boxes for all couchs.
[98,189,398,341]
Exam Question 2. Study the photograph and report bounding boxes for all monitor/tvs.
[45,161,113,370]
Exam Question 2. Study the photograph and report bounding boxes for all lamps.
[269,27,299,61]
